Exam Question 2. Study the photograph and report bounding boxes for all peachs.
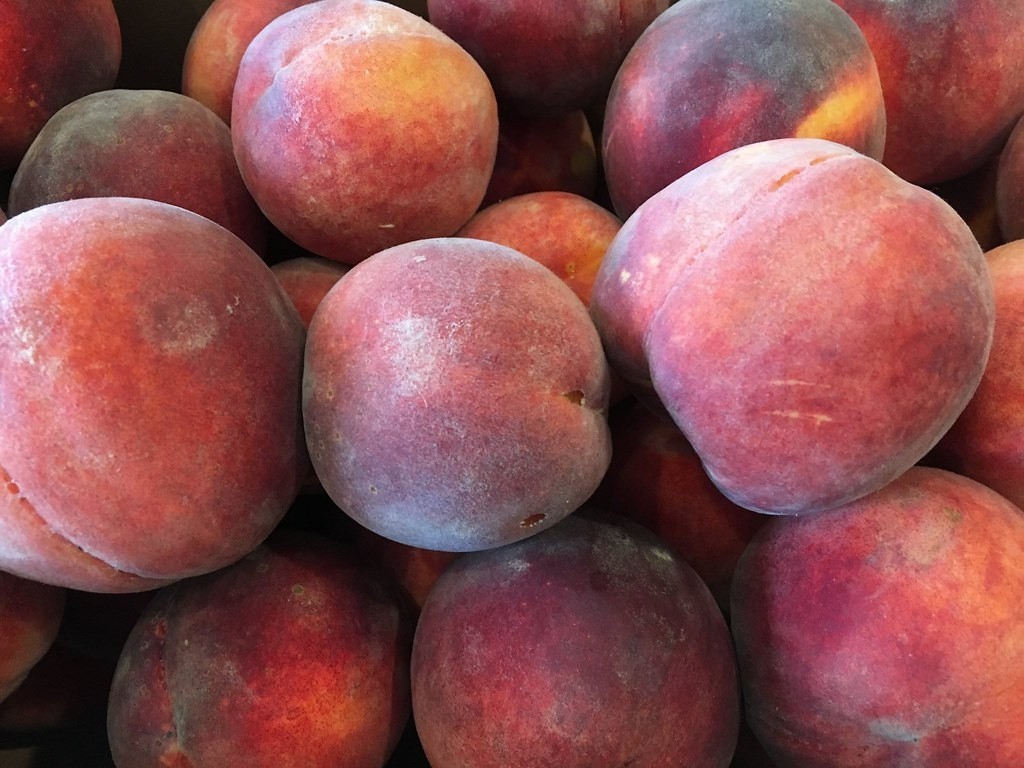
[0,0,1024,768]
